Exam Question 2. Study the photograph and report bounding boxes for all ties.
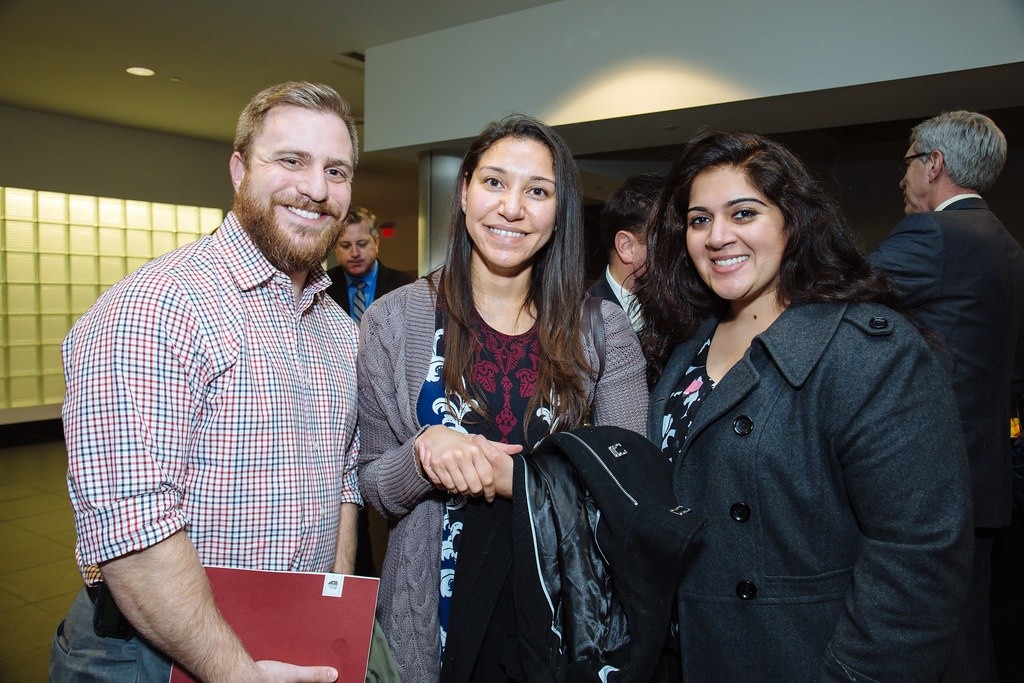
[353,279,368,326]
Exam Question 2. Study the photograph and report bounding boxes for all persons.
[325,206,415,327]
[646,128,999,683]
[870,110,1023,683]
[356,109,651,683]
[585,173,669,339]
[49,81,363,681]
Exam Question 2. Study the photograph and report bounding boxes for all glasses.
[898,152,930,175]
